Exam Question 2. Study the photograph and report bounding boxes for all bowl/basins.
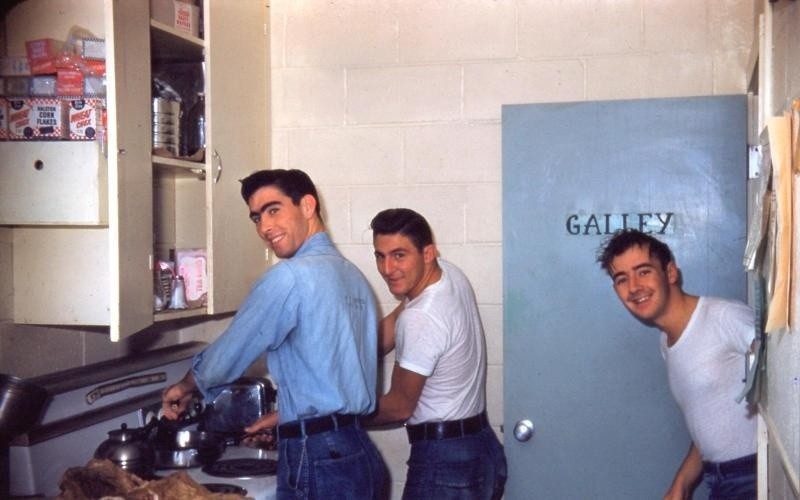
[164,445,202,468]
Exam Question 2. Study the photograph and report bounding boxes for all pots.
[155,427,274,471]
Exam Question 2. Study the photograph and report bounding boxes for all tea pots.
[92,422,157,481]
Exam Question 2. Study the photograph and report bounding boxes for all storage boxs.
[151,0,201,38]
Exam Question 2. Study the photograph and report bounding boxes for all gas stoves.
[154,444,279,499]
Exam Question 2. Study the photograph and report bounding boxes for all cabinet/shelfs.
[0,0,272,342]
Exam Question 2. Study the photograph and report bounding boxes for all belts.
[405,410,492,444]
[270,407,359,439]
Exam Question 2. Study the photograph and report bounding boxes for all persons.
[363,208,508,500]
[595,229,756,500]
[163,169,393,500]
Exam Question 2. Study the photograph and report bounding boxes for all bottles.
[166,275,189,310]
[153,259,165,313]
[188,92,205,161]
